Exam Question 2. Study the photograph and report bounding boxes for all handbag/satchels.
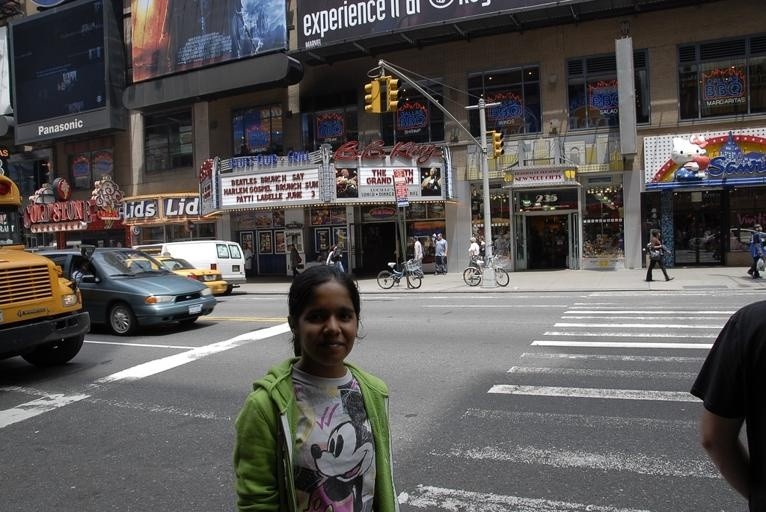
[650,250,660,260]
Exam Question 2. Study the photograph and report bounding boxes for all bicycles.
[462,254,511,287]
[376,259,424,290]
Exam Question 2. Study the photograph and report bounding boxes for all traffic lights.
[493,132,504,156]
[364,79,381,114]
[387,76,402,112]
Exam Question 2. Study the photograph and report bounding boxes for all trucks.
[129,239,247,294]
[1,174,96,369]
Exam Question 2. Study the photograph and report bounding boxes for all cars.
[406,235,432,252]
[32,247,217,335]
[689,228,766,259]
[123,256,230,295]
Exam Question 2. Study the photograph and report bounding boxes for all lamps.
[501,171,514,183]
[564,166,577,184]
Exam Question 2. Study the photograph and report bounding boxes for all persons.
[243,243,253,277]
[71,262,94,281]
[232,266,400,512]
[318,232,329,249]
[748,224,766,279]
[690,301,766,512]
[712,233,720,259]
[478,236,485,272]
[646,230,674,281]
[414,236,424,278]
[422,167,440,190]
[338,230,346,248]
[326,244,339,267]
[290,244,302,279]
[336,169,356,186]
[432,233,448,275]
[469,237,480,280]
